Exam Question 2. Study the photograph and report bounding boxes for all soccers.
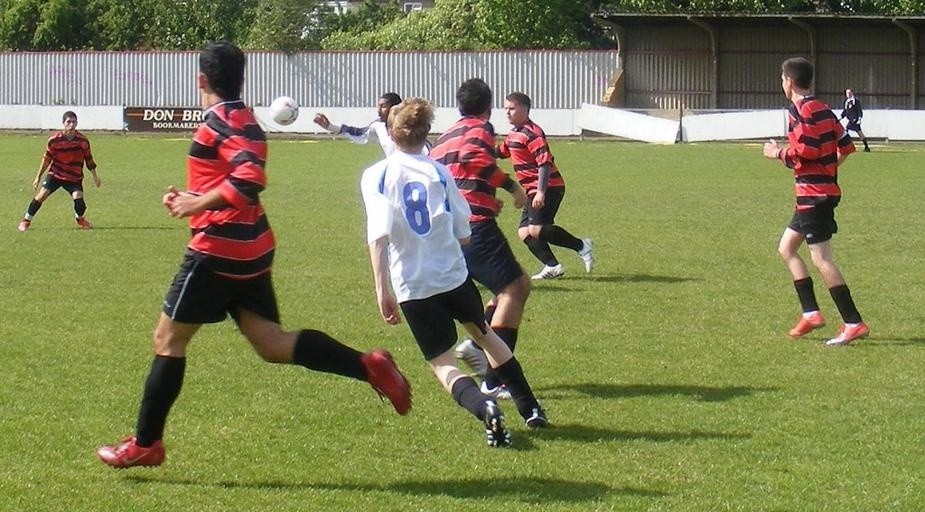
[270,95,299,126]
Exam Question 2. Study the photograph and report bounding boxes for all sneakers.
[529,264,563,280]
[789,312,824,336]
[362,348,413,415]
[76,215,92,228]
[865,146,870,152]
[524,408,549,426]
[579,239,595,273]
[99,434,165,470]
[19,218,31,232]
[486,403,510,446]
[827,323,870,345]
[456,339,511,399]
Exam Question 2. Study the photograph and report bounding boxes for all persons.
[94,37,414,469]
[426,78,528,401]
[762,55,871,347]
[311,91,434,161]
[17,111,101,231]
[494,91,594,280]
[359,95,548,449]
[838,87,871,153]
